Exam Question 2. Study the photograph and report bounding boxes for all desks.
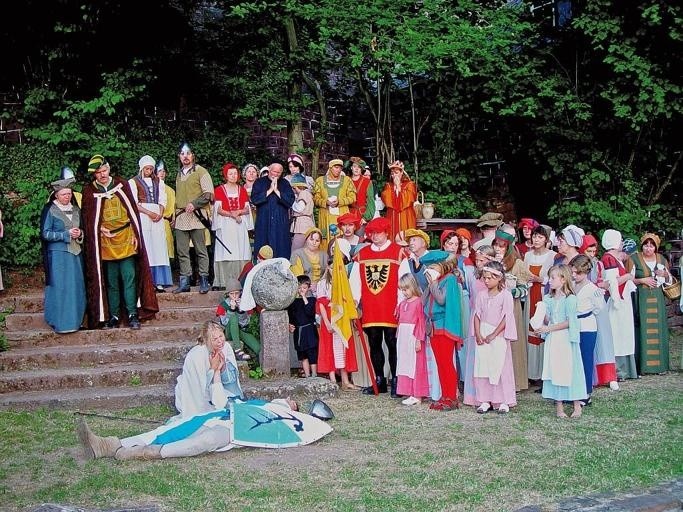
[416,218,479,234]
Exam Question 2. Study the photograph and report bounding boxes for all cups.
[421,203,434,219]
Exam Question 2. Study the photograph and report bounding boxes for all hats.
[259,166,269,177]
[221,279,244,296]
[406,213,661,249]
[241,163,259,179]
[345,155,373,173]
[137,155,155,171]
[289,172,307,189]
[222,162,240,182]
[386,160,406,172]
[85,154,108,176]
[47,177,75,192]
[256,244,275,261]
[285,153,305,167]
[328,158,344,168]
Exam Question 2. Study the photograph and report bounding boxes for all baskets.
[661,275,681,300]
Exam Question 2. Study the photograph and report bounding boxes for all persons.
[127,157,174,293]
[80,154,159,330]
[175,318,244,412]
[66,395,302,461]
[0,209,5,295]
[38,176,88,335]
[172,143,214,295]
[154,159,176,259]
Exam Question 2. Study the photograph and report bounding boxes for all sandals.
[578,396,592,408]
[475,402,492,413]
[340,382,360,391]
[497,403,510,413]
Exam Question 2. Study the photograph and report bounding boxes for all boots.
[77,416,121,464]
[361,375,387,395]
[390,376,402,399]
[171,275,191,293]
[196,274,209,294]
[111,442,166,466]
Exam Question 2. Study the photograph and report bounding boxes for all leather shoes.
[98,315,119,329]
[127,314,140,330]
[155,286,164,292]
[140,312,157,320]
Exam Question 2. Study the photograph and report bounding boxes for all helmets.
[154,159,168,173]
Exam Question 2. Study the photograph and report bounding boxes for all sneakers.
[608,380,620,391]
[401,395,421,407]
[427,395,459,413]
